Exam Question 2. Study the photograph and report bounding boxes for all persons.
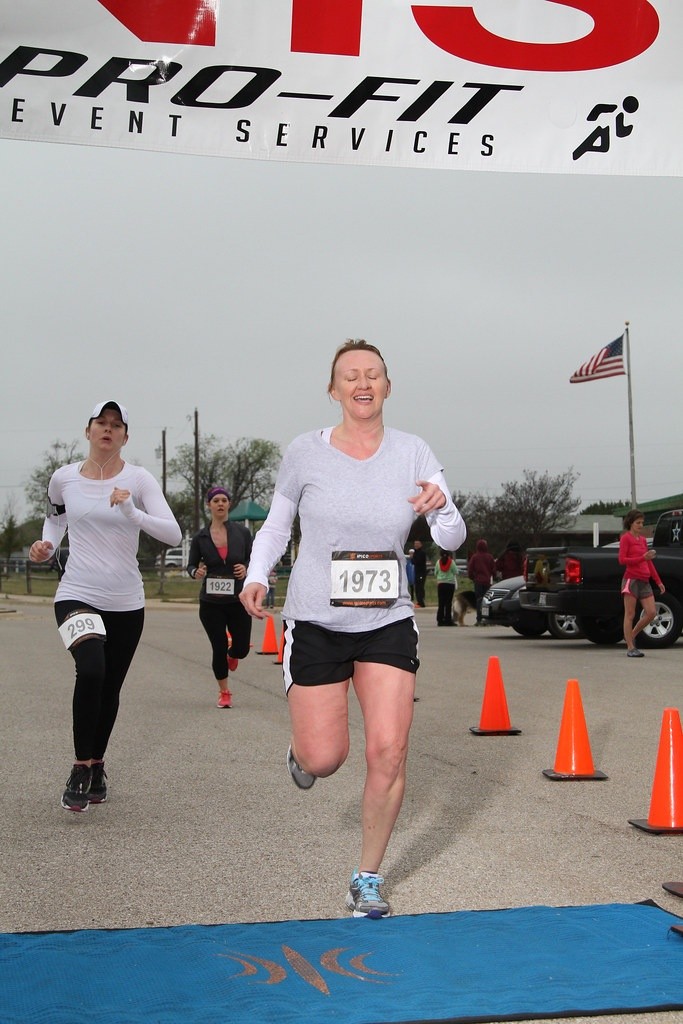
[266,571,278,610]
[411,539,427,608]
[239,336,466,918]
[28,400,182,812]
[468,540,497,626]
[618,510,665,657]
[496,537,529,580]
[185,486,253,708]
[434,551,459,626]
[406,549,415,600]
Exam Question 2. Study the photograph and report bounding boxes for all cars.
[481,538,655,639]
[455,559,468,573]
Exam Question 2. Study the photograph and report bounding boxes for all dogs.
[451,590,477,626]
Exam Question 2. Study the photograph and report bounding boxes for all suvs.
[155,548,182,568]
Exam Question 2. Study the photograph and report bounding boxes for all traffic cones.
[227,633,232,645]
[542,678,609,781]
[627,708,683,833]
[273,622,284,665]
[256,614,279,655]
[469,655,522,735]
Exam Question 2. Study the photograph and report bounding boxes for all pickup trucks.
[518,508,683,648]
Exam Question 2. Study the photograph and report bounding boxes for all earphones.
[124,438,127,443]
[86,433,90,439]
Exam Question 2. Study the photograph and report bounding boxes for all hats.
[87,400,129,429]
[207,487,231,504]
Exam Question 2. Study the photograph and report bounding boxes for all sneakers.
[227,645,238,671]
[635,647,640,652]
[344,871,390,918]
[61,764,89,812]
[287,744,316,790]
[217,691,232,708]
[627,648,645,657]
[87,761,108,803]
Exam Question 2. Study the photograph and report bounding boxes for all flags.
[570,334,627,384]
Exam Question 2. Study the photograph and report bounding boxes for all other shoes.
[437,621,444,627]
[414,603,421,608]
[444,619,458,627]
